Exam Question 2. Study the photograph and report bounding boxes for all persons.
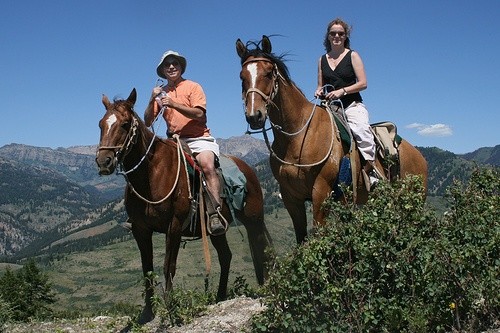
[314,18,380,192]
[144,50,226,235]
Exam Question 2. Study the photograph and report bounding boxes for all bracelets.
[343,87,348,96]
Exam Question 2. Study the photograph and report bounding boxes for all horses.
[96,88,273,326]
[236,34,428,255]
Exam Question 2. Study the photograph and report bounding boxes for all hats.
[155,50,187,79]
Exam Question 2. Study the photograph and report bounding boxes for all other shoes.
[368,171,381,190]
[208,212,225,234]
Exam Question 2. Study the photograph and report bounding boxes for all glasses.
[164,61,180,67]
[328,32,345,36]
